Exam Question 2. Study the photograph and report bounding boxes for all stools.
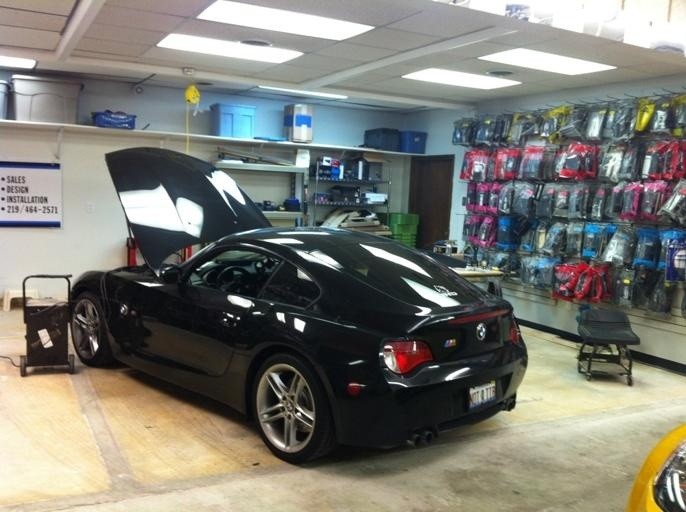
[1,285,41,314]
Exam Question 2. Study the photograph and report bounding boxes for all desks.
[453,265,504,295]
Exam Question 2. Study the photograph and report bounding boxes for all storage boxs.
[9,74,85,124]
[400,131,427,152]
[364,128,400,150]
[209,103,257,138]
[1,79,10,118]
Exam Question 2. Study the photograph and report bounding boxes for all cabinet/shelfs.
[213,160,309,227]
[309,157,392,231]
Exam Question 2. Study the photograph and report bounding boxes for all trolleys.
[20,272,76,376]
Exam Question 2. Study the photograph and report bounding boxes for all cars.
[69,146,529,467]
[626,421,686,511]
